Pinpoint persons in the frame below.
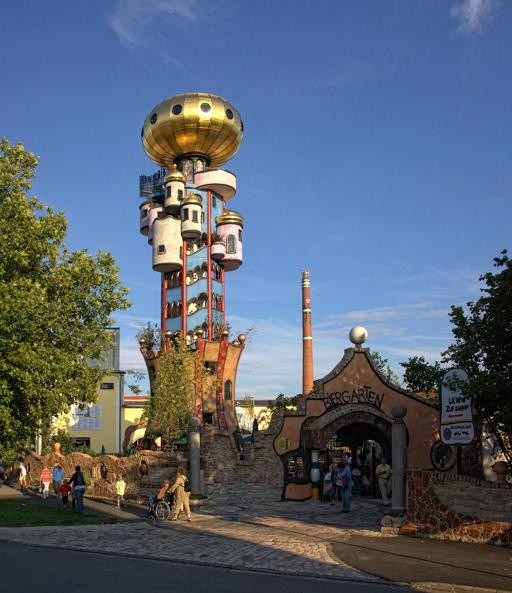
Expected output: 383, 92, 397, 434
126, 440, 144, 455
115, 474, 128, 509
141, 479, 170, 520
0, 462, 27, 491
166, 465, 192, 523
39, 464, 86, 513
331, 446, 392, 512
101, 443, 107, 456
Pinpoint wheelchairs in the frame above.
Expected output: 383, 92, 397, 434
148, 491, 173, 521
